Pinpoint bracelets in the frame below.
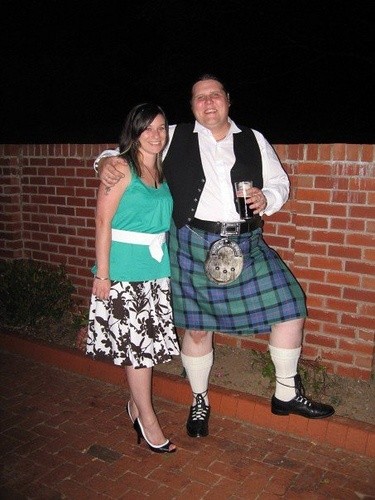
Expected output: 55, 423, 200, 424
95, 275, 110, 280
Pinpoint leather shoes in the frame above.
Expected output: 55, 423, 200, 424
186, 388, 212, 439
270, 395, 336, 419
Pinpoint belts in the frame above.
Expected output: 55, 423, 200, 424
188, 214, 267, 235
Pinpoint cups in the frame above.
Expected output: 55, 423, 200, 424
235, 181, 254, 220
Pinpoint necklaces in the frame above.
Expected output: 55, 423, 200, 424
144, 164, 157, 188
216, 135, 226, 142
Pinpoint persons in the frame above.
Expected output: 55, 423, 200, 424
86, 103, 181, 454
91, 69, 336, 440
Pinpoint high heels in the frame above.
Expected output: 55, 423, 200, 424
126, 400, 134, 424
132, 416, 178, 455
